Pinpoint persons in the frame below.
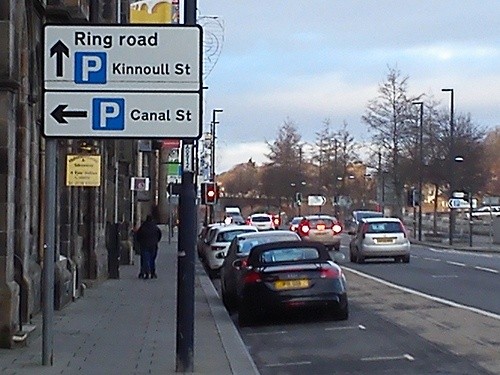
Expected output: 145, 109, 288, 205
133, 216, 162, 280
107, 220, 135, 276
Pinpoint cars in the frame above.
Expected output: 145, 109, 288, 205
297, 215, 342, 250
197, 207, 302, 279
232, 240, 349, 321
465, 206, 500, 218
288, 217, 303, 232
344, 210, 383, 234
215, 230, 305, 313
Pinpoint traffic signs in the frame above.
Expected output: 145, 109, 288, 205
42, 22, 204, 92
42, 90, 203, 141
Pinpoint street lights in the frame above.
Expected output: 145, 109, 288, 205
348, 217, 411, 264
210, 109, 223, 181
412, 101, 424, 242
441, 87, 455, 248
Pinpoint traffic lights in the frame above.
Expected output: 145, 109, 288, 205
200, 182, 219, 206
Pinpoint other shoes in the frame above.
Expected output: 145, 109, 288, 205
151, 273, 155, 279
144, 273, 150, 280
